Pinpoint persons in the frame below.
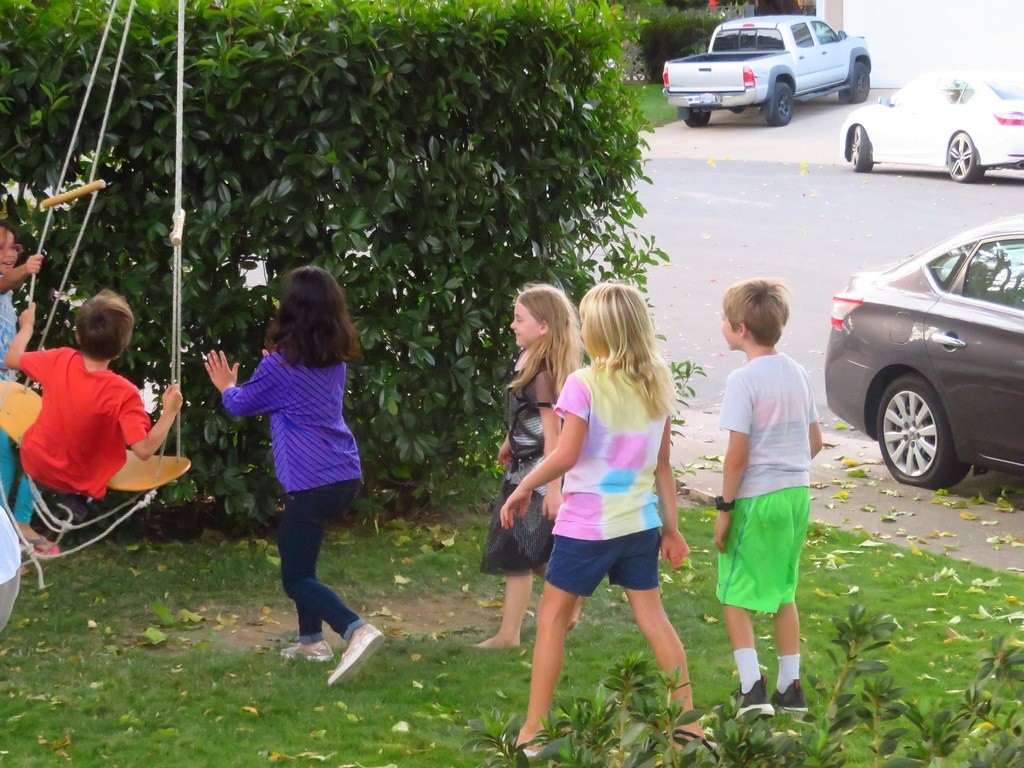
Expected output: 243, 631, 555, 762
205, 267, 385, 685
0, 221, 182, 633
712, 280, 822, 725
472, 283, 716, 768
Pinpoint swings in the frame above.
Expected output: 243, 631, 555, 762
0, 0, 192, 590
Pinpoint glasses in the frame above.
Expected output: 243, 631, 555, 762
0, 244, 24, 255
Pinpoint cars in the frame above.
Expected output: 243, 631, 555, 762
837, 62, 1024, 185
822, 212, 1023, 492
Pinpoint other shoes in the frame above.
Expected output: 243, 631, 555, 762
511, 737, 539, 758
697, 741, 717, 749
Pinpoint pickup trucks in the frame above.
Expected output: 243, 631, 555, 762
660, 13, 873, 128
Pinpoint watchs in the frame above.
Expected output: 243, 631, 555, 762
715, 495, 737, 512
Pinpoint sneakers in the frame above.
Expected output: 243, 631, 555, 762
730, 675, 775, 722
327, 624, 385, 686
769, 679, 808, 712
281, 640, 334, 662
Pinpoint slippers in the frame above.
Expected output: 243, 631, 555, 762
20, 533, 59, 556
